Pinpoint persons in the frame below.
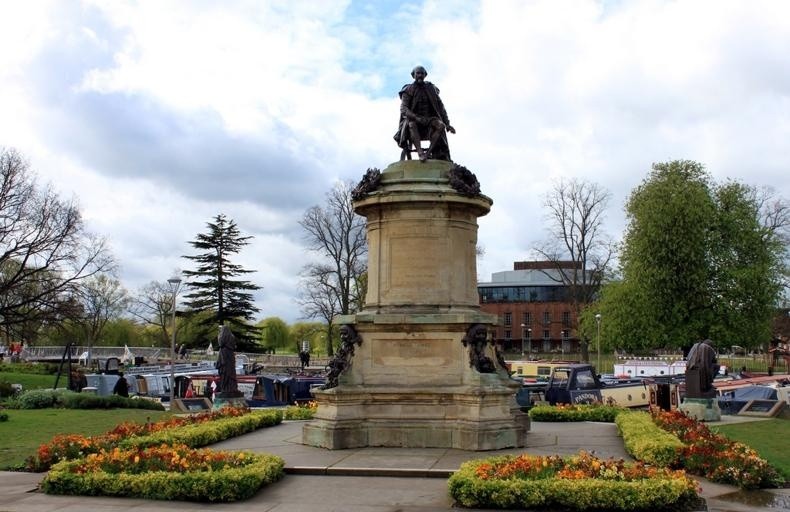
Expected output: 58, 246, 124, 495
175, 343, 179, 359
179, 344, 187, 360
215, 325, 239, 394
69, 367, 87, 393
686, 339, 720, 393
112, 372, 129, 397
299, 350, 311, 372
392, 66, 456, 163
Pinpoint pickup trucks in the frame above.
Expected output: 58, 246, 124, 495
545, 365, 658, 408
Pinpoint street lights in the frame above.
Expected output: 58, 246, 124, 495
167, 276, 182, 413
595, 314, 602, 377
520, 324, 532, 357
560, 332, 565, 360
